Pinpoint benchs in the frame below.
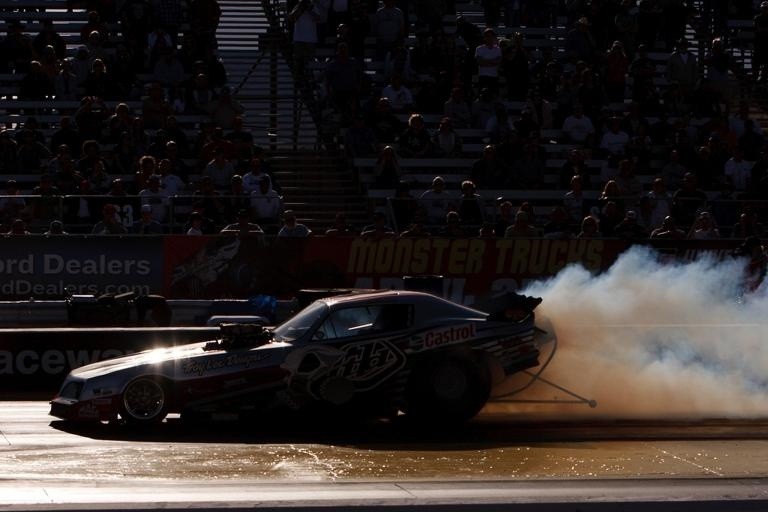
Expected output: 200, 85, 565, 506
1, 0, 288, 232
293, 0, 767, 241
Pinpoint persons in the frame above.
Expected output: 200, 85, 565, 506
1, 0, 765, 242
727, 235, 767, 292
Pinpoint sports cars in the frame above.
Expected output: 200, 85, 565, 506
48, 289, 547, 444
